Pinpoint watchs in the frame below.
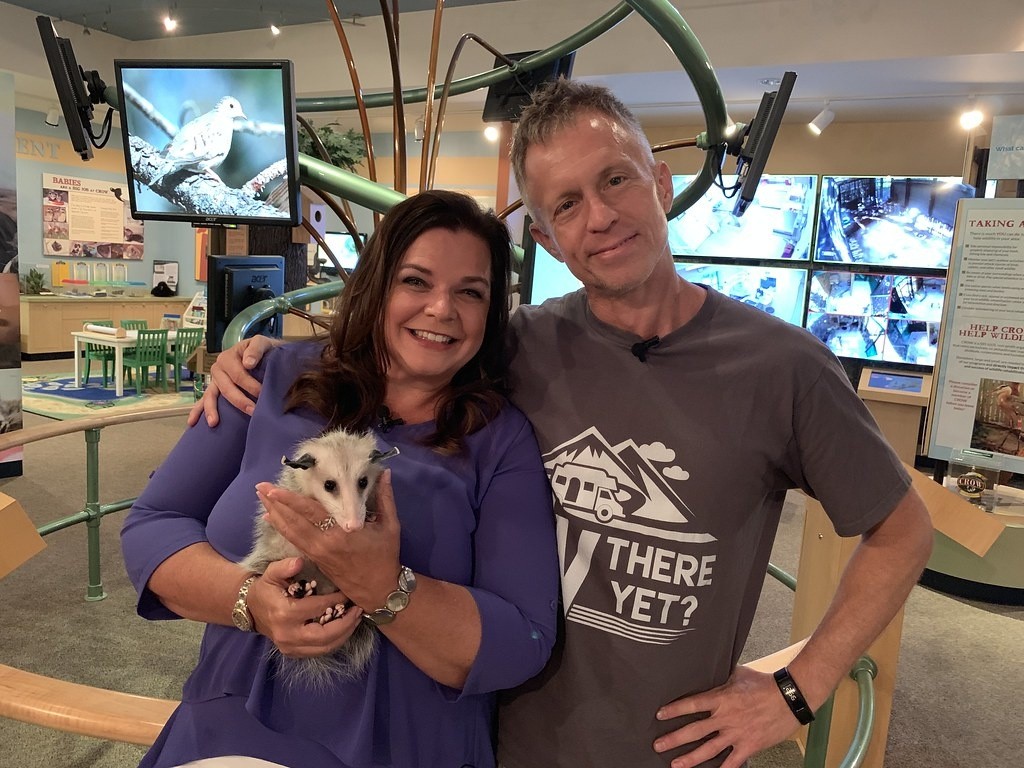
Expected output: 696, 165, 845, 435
229, 574, 259, 635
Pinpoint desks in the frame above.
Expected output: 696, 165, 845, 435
71, 329, 205, 396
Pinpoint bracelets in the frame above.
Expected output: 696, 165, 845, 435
358, 563, 417, 626
773, 667, 816, 727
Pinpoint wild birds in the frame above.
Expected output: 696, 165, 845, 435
148, 96, 247, 189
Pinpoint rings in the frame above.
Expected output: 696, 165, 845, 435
311, 513, 336, 532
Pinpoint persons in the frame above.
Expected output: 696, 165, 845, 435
117, 189, 560, 768
43, 189, 67, 206
185, 82, 934, 768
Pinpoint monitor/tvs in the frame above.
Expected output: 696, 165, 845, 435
318, 232, 366, 272
116, 59, 300, 221
663, 175, 966, 371
36, 15, 90, 161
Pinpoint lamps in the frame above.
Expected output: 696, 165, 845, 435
414, 115, 424, 140
102, 22, 108, 32
726, 114, 736, 135
270, 12, 287, 34
808, 100, 834, 134
82, 15, 90, 35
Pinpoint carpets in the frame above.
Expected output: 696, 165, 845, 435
22, 364, 195, 421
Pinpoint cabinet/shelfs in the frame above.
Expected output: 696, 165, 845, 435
20, 299, 192, 353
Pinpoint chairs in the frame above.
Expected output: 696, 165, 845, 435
84, 320, 204, 396
